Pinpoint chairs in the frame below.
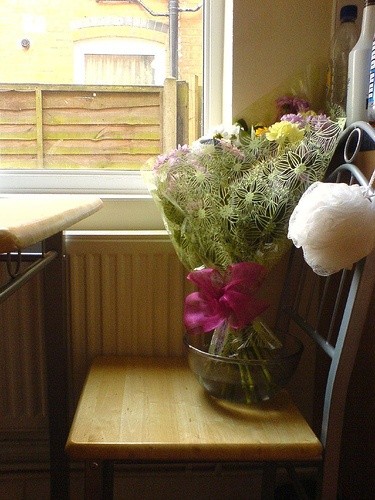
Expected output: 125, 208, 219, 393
64, 118, 375, 500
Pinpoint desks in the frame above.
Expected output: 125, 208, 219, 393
0, 192, 103, 500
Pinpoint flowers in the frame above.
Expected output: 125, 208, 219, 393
140, 56, 347, 411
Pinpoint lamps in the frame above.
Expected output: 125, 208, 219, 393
331, 8, 360, 68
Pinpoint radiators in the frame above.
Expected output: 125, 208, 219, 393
0, 229, 217, 431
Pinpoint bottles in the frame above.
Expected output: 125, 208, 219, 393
348, 3, 374, 127
327, 6, 358, 125
368, 41, 375, 123
